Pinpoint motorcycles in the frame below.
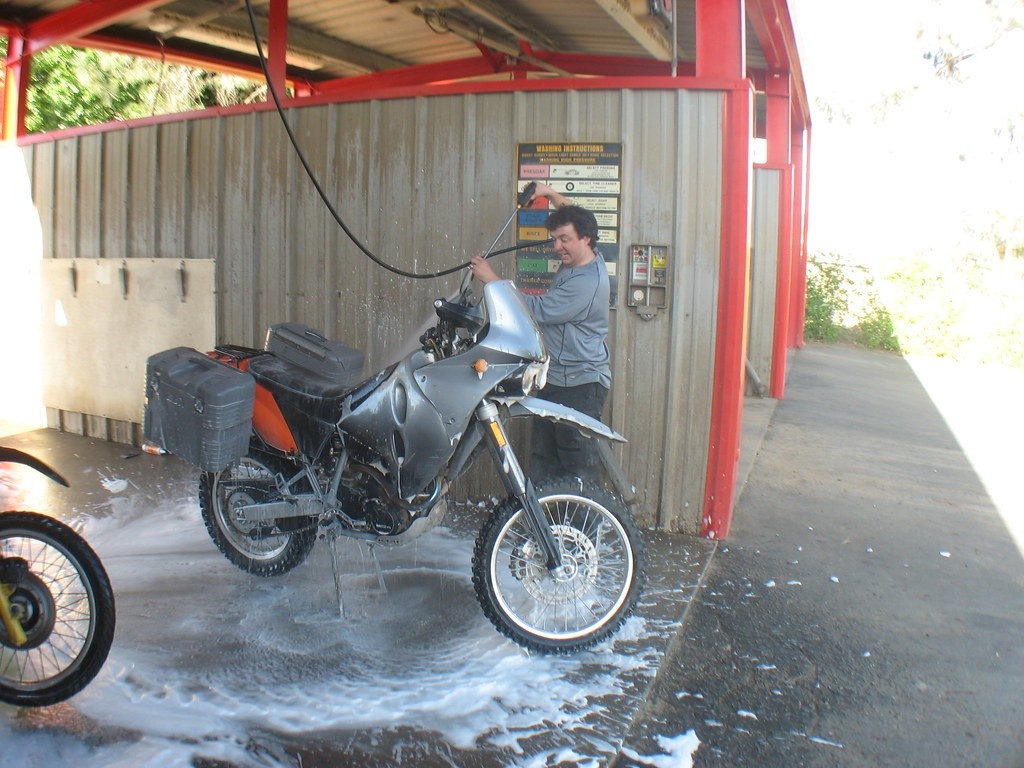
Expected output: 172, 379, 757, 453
143, 281, 647, 658
0, 447, 116, 706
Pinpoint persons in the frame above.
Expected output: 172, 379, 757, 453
470, 179, 612, 565
